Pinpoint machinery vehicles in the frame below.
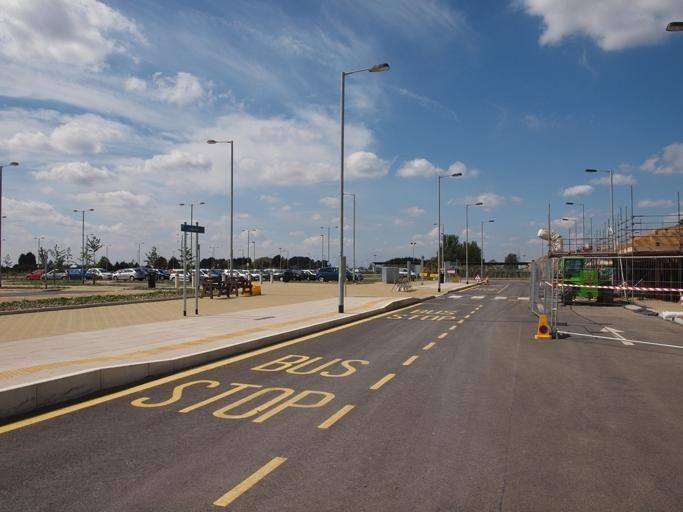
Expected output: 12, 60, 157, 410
559, 257, 616, 305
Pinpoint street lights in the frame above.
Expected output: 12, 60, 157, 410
206, 139, 235, 281
561, 168, 616, 254
73, 209, 94, 274
436, 172, 495, 292
179, 202, 205, 275
338, 63, 390, 313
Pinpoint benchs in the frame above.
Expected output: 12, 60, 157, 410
200, 275, 253, 299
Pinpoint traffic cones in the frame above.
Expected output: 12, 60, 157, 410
534, 314, 552, 341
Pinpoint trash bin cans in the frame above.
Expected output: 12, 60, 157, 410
148, 276, 156, 289
440, 274, 444, 283
